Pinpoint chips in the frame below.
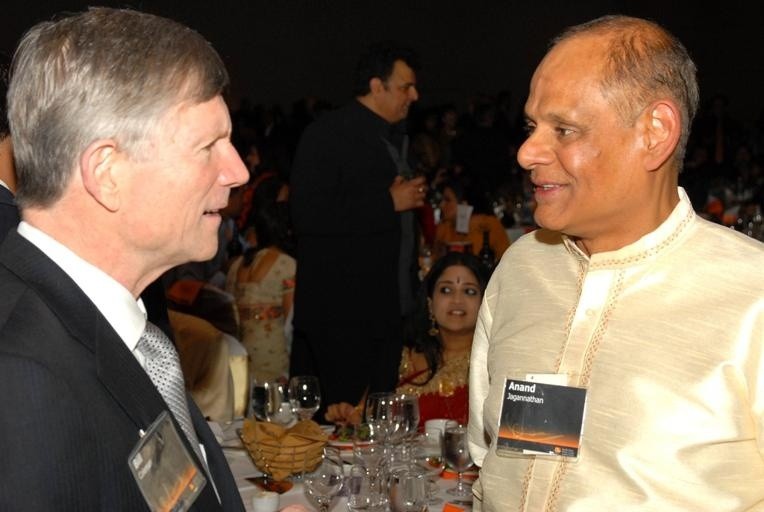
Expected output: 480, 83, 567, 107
242, 418, 329, 482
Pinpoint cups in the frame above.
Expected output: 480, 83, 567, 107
252, 373, 471, 511
397, 165, 422, 184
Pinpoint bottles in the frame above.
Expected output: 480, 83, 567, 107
479, 231, 495, 265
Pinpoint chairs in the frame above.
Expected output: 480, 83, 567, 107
192, 285, 240, 339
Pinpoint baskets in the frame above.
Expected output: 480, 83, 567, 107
236, 428, 326, 480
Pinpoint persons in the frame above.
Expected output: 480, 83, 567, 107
1, 88, 349, 389
391, 90, 763, 441
469, 15, 763, 511
288, 42, 430, 435
0, 5, 252, 511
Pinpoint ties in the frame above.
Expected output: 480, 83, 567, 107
137, 320, 221, 505
